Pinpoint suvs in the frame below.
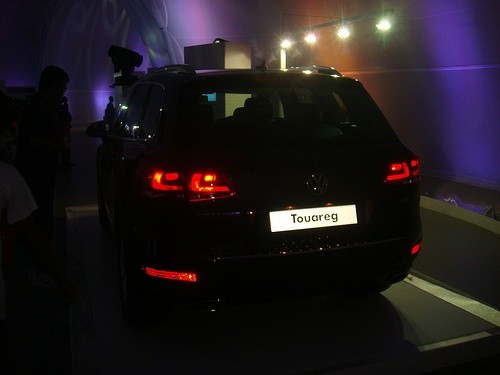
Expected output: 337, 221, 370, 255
84, 64, 423, 325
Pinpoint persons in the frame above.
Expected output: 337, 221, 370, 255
104, 96, 115, 131
0, 162, 42, 319
0, 65, 72, 242
